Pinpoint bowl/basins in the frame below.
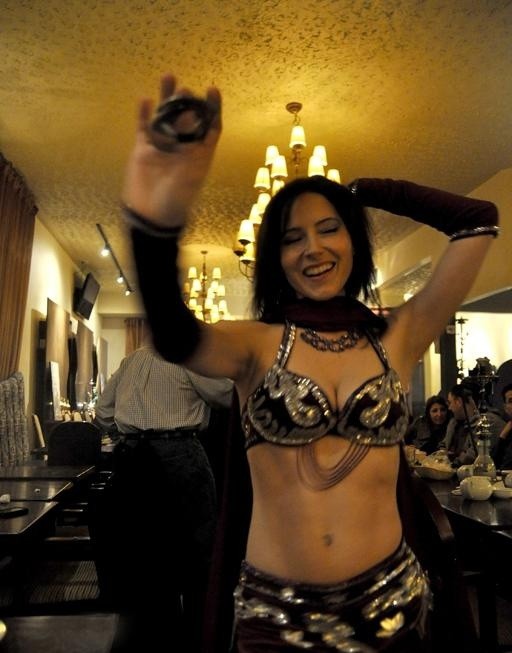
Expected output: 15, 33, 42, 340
490, 486, 511, 498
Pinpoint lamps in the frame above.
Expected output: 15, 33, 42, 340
234, 102, 341, 287
95, 223, 132, 296
182, 250, 233, 326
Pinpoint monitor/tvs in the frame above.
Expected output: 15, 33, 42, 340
74, 272, 101, 320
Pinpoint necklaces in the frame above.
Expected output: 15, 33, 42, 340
301, 326, 365, 354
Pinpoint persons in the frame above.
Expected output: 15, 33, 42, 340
401, 372, 512, 474
92, 344, 235, 652
122, 73, 496, 651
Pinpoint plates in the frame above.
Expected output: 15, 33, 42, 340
412, 465, 455, 479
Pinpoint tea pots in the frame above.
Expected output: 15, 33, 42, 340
459, 476, 493, 502
